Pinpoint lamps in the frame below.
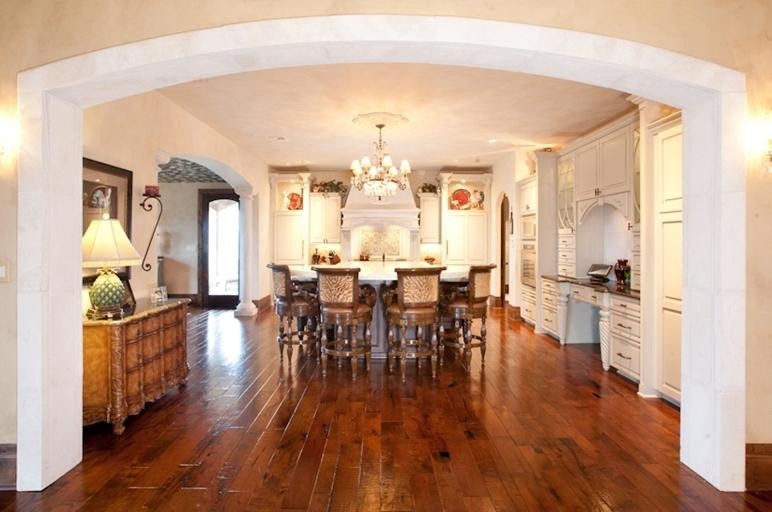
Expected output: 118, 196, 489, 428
83, 217, 144, 321
348, 121, 412, 201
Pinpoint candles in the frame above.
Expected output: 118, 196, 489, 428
144, 185, 161, 195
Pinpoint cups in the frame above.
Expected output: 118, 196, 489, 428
152, 286, 167, 304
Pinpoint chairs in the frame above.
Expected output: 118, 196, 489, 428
266, 263, 497, 383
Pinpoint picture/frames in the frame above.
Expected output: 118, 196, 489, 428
83, 157, 132, 282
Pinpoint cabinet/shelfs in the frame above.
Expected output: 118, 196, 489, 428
310, 192, 341, 245
269, 171, 309, 264
440, 170, 489, 261
416, 193, 440, 245
83, 297, 194, 435
517, 115, 641, 384
